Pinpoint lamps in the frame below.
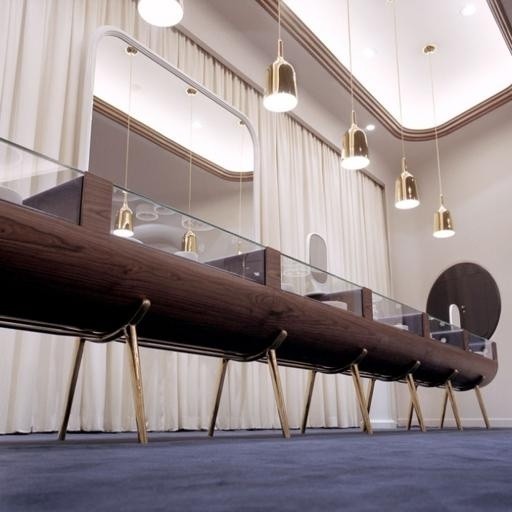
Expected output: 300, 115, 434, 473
235, 121, 248, 259
264, 0, 298, 113
393, 1, 420, 209
136, 0, 184, 28
426, 44, 458, 239
341, 1, 369, 172
114, 47, 139, 238
181, 89, 199, 259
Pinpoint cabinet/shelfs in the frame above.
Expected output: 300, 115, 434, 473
0, 139, 498, 443
